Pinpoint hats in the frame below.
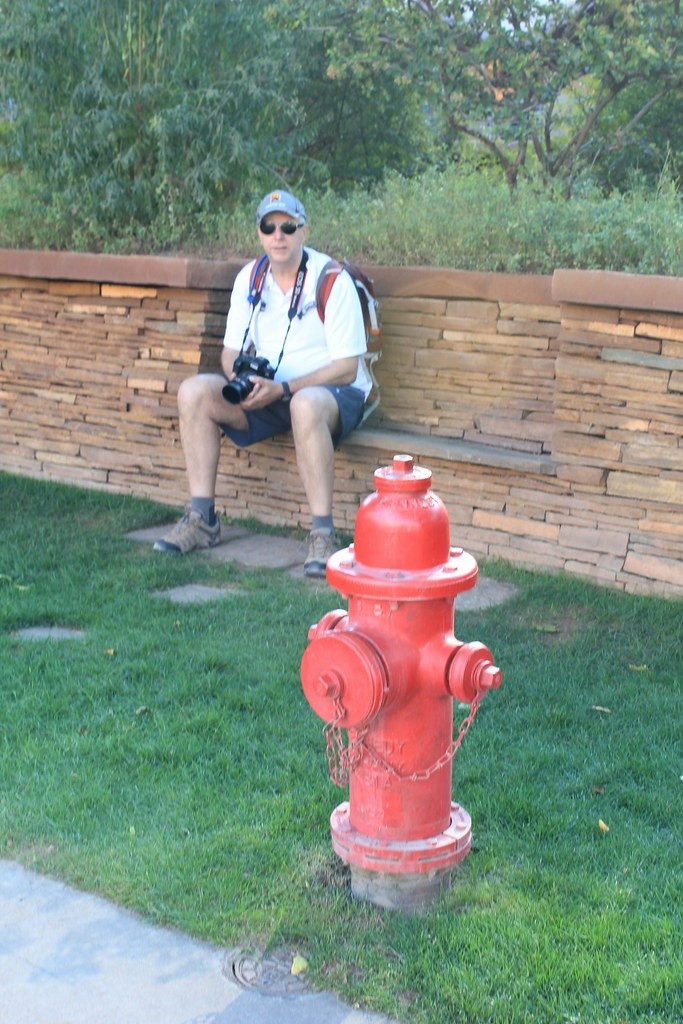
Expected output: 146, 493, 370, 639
255, 189, 306, 225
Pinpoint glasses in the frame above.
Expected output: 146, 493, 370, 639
260, 221, 305, 235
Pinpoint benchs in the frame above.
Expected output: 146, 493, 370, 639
290, 423, 558, 575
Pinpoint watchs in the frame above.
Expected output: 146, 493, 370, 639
281, 381, 293, 402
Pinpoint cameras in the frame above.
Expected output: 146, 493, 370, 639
221, 354, 274, 405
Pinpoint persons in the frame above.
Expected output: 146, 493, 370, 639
152, 188, 374, 578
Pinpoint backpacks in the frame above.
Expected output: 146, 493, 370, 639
248, 254, 382, 364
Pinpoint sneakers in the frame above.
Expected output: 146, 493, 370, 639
296, 527, 343, 578
152, 506, 222, 553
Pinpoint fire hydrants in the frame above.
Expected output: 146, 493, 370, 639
297, 452, 503, 921
